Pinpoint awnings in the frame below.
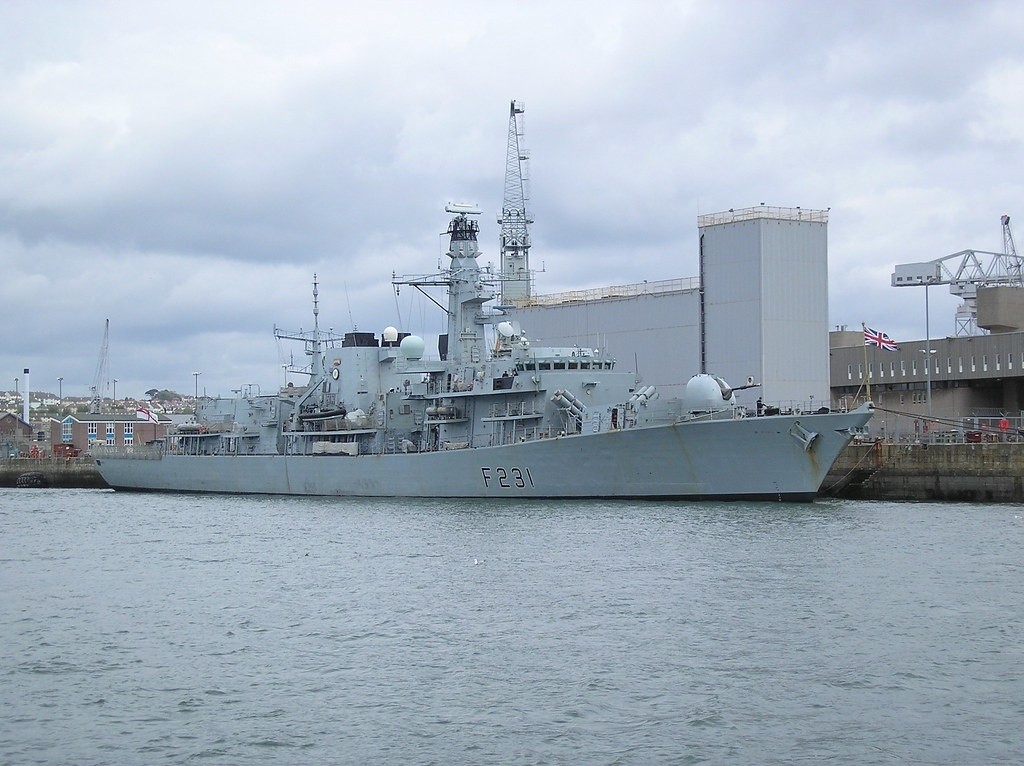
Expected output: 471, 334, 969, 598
863, 327, 897, 352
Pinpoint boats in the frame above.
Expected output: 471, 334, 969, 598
93, 205, 876, 507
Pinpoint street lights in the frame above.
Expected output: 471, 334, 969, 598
112, 378, 118, 403
14, 377, 19, 430
192, 371, 202, 399
57, 377, 64, 422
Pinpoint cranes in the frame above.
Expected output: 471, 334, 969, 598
890, 215, 1024, 339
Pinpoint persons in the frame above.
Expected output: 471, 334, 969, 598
502, 371, 508, 377
757, 397, 763, 417
